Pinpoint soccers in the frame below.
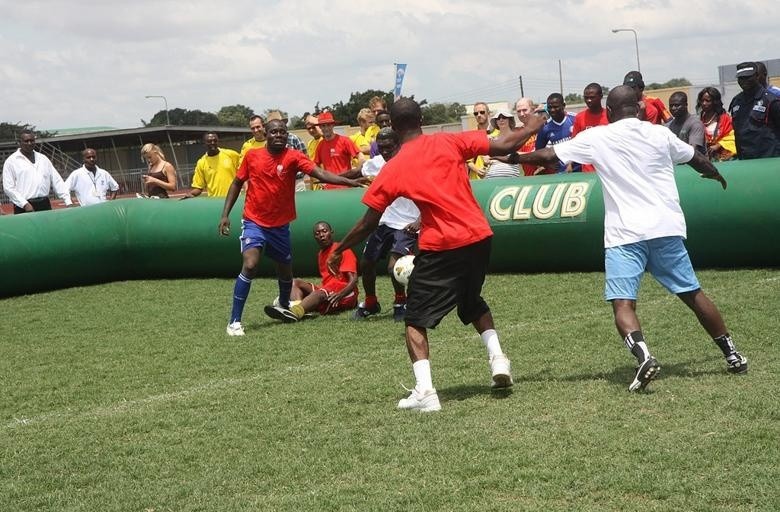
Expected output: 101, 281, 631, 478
393, 253, 415, 284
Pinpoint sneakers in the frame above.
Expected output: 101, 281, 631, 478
726, 355, 748, 375
626, 355, 661, 393
263, 305, 298, 324
392, 300, 407, 323
226, 320, 246, 337
351, 302, 381, 322
397, 386, 441, 413
488, 357, 514, 390
273, 296, 292, 310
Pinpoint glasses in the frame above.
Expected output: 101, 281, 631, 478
473, 111, 487, 115
495, 114, 509, 120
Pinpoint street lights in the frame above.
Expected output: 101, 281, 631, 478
145, 95, 170, 126
611, 28, 641, 72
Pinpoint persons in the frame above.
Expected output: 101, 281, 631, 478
623, 62, 780, 165
181, 95, 392, 200
2, 130, 64, 214
326, 97, 549, 412
65, 147, 120, 208
264, 220, 360, 322
140, 143, 178, 199
218, 118, 371, 336
488, 84, 748, 391
310, 125, 420, 322
465, 83, 610, 179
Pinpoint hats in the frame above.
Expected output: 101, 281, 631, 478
734, 61, 758, 78
489, 108, 517, 130
312, 112, 338, 127
263, 109, 289, 126
622, 71, 642, 89
